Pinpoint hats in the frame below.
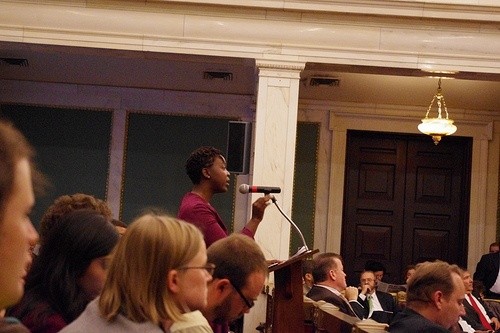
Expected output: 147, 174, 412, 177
467, 293, 495, 333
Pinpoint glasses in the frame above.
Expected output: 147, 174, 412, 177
176, 263, 216, 276
229, 275, 255, 309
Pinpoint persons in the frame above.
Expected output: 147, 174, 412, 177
0, 121, 500, 333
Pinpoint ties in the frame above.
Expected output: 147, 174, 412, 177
339, 294, 359, 318
368, 296, 373, 313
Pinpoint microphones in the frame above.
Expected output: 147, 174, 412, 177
238, 184, 282, 197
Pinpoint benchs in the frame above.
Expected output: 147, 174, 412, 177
261, 283, 317, 333
315, 302, 389, 333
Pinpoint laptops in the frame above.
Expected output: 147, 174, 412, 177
369, 310, 394, 325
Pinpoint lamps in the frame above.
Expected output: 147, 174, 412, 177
417, 79, 457, 145
